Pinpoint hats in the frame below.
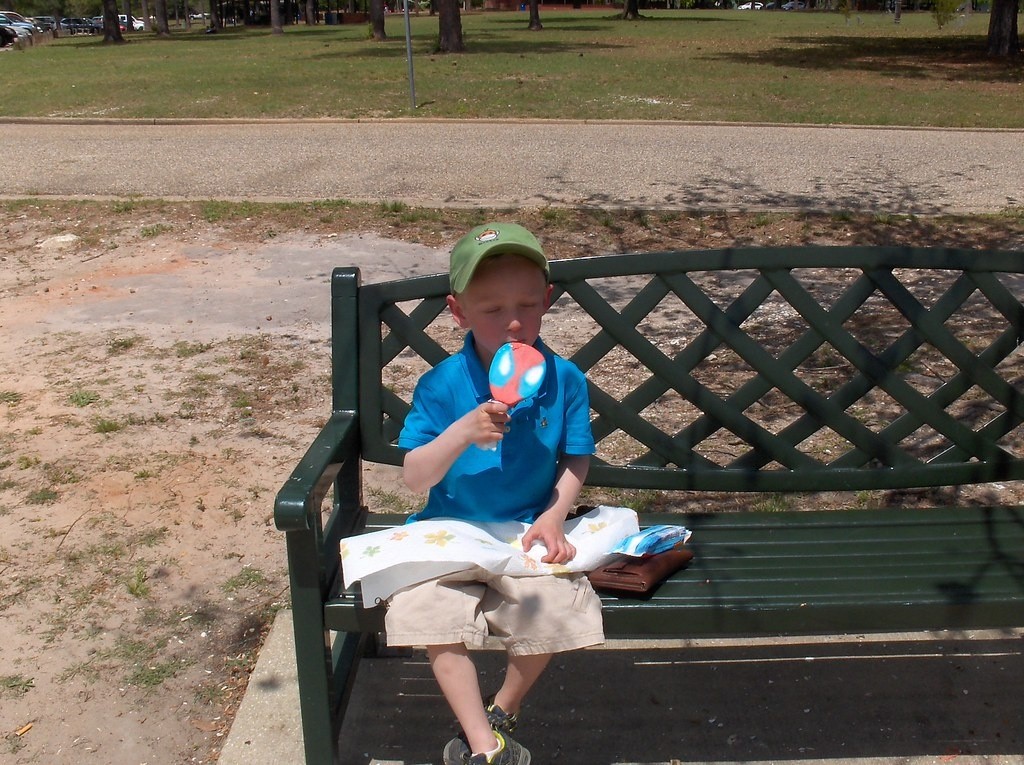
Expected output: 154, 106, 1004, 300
449, 222, 550, 296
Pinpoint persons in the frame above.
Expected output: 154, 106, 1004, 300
520, 3, 525, 11
384, 6, 388, 13
386, 222, 604, 765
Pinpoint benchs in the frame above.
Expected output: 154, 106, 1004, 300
272, 243, 1023, 765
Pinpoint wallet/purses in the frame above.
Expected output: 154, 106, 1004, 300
588, 548, 693, 592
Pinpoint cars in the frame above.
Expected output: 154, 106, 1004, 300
188, 12, 210, 20
738, 1, 764, 10
760, 2, 782, 10
0, 10, 157, 48
780, 2, 805, 12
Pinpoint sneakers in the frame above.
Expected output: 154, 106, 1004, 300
443, 704, 519, 765
458, 728, 532, 765
